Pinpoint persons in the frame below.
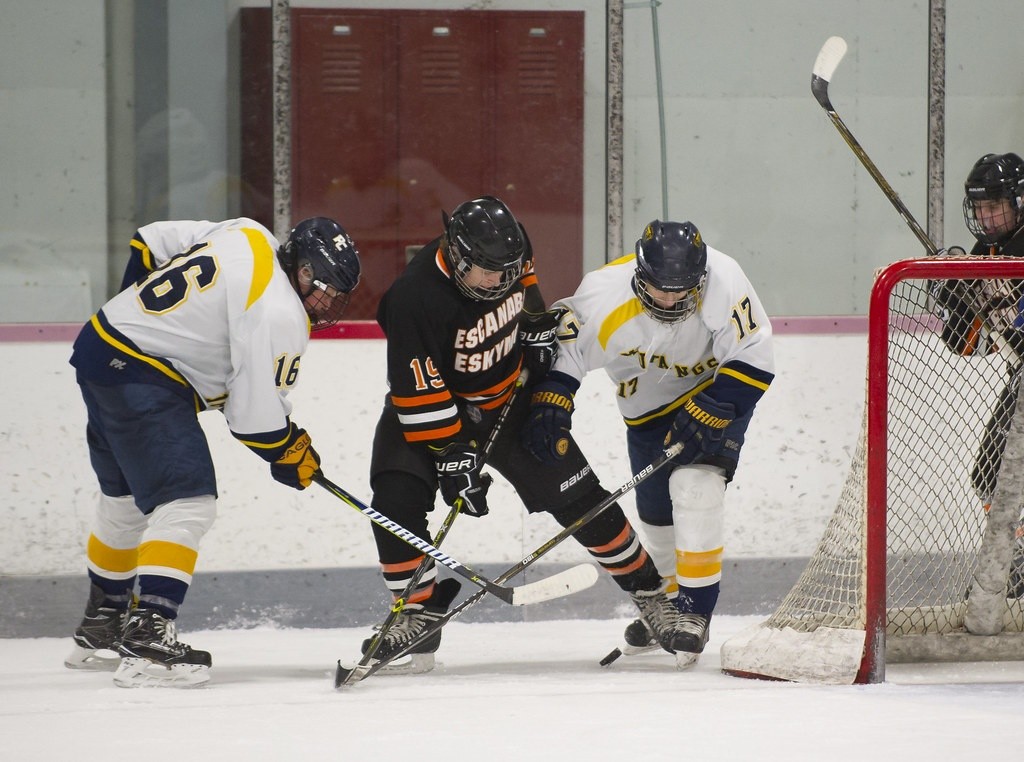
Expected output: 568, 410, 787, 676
67, 215, 360, 691
522, 219, 775, 670
928, 153, 1024, 634
362, 195, 679, 676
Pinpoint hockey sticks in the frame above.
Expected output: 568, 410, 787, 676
810, 34, 1022, 371
334, 432, 683, 685
310, 475, 600, 608
334, 364, 535, 692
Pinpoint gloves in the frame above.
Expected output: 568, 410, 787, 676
429, 446, 492, 516
941, 310, 995, 356
924, 246, 985, 305
526, 408, 572, 462
664, 396, 735, 465
272, 429, 320, 492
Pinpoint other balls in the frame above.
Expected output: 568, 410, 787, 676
599, 646, 622, 667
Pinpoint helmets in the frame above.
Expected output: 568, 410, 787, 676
288, 216, 361, 289
966, 152, 1023, 198
450, 195, 527, 270
634, 221, 706, 290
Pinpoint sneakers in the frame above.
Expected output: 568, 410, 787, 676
63, 579, 212, 685
675, 588, 711, 666
627, 582, 682, 656
623, 610, 656, 656
361, 579, 461, 676
